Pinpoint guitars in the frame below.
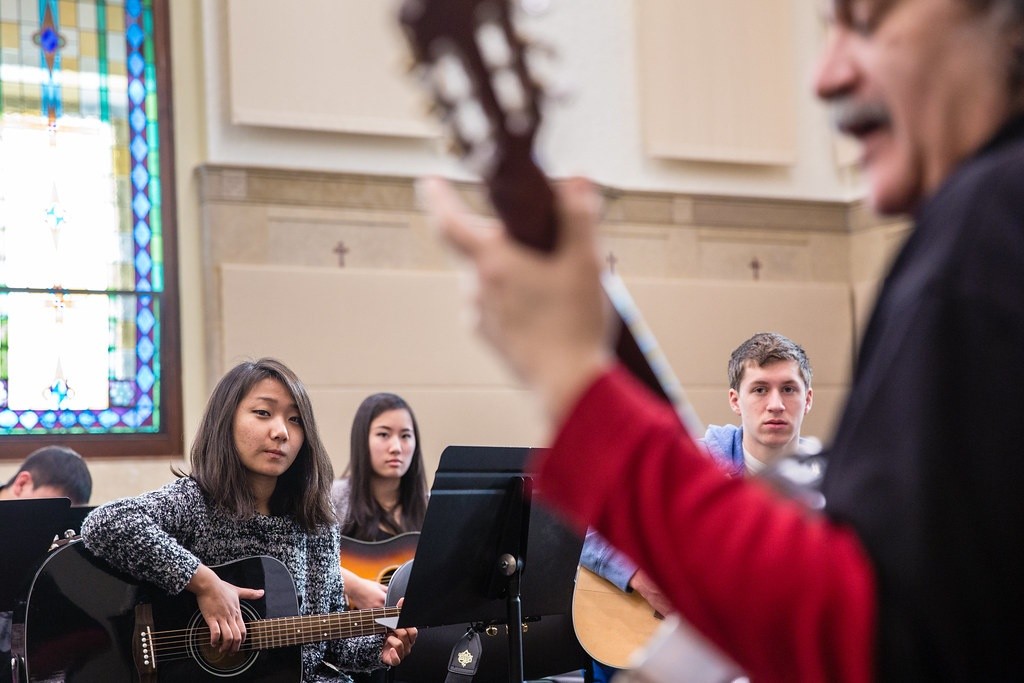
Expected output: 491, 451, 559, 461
340, 531, 423, 611
9, 528, 404, 683
396, 0, 688, 427
570, 560, 669, 670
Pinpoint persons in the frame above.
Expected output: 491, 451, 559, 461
0, 445, 92, 683
80, 357, 418, 683
332, 392, 431, 610
418, 1, 1023, 681
580, 334, 830, 622
